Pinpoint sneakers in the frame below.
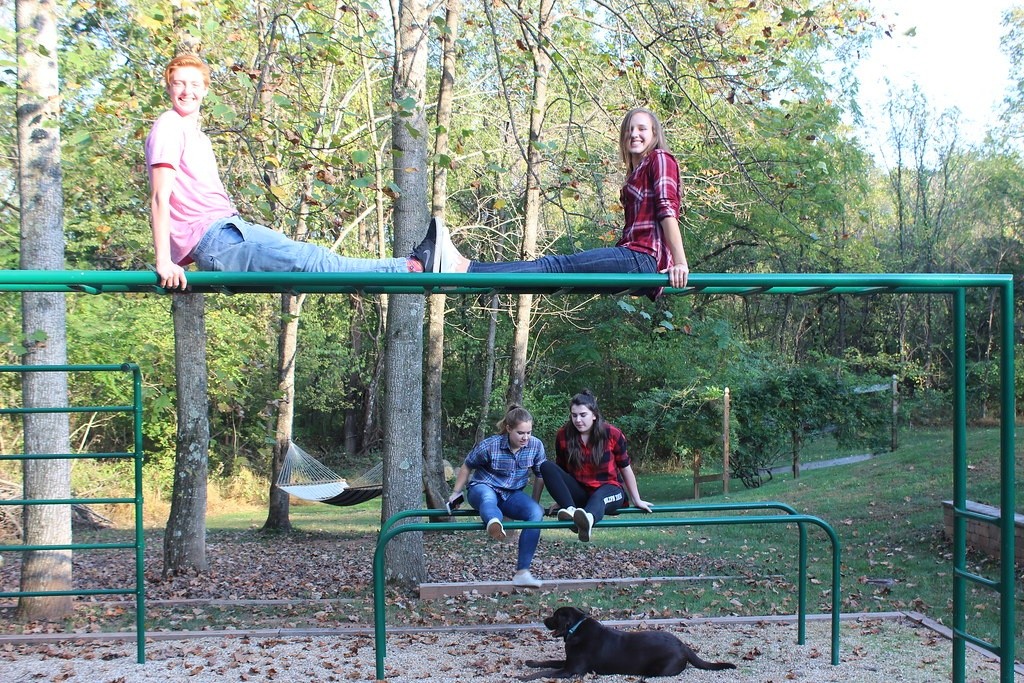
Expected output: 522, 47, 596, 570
410, 217, 443, 274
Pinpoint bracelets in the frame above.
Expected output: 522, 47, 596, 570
453, 490, 461, 494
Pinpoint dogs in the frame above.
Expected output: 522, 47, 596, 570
518, 607, 738, 683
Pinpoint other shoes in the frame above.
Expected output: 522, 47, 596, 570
558, 507, 578, 534
486, 518, 507, 542
439, 226, 461, 273
512, 571, 542, 588
575, 508, 593, 542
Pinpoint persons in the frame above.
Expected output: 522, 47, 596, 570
440, 108, 691, 290
145, 55, 442, 291
540, 388, 655, 542
449, 402, 547, 588
